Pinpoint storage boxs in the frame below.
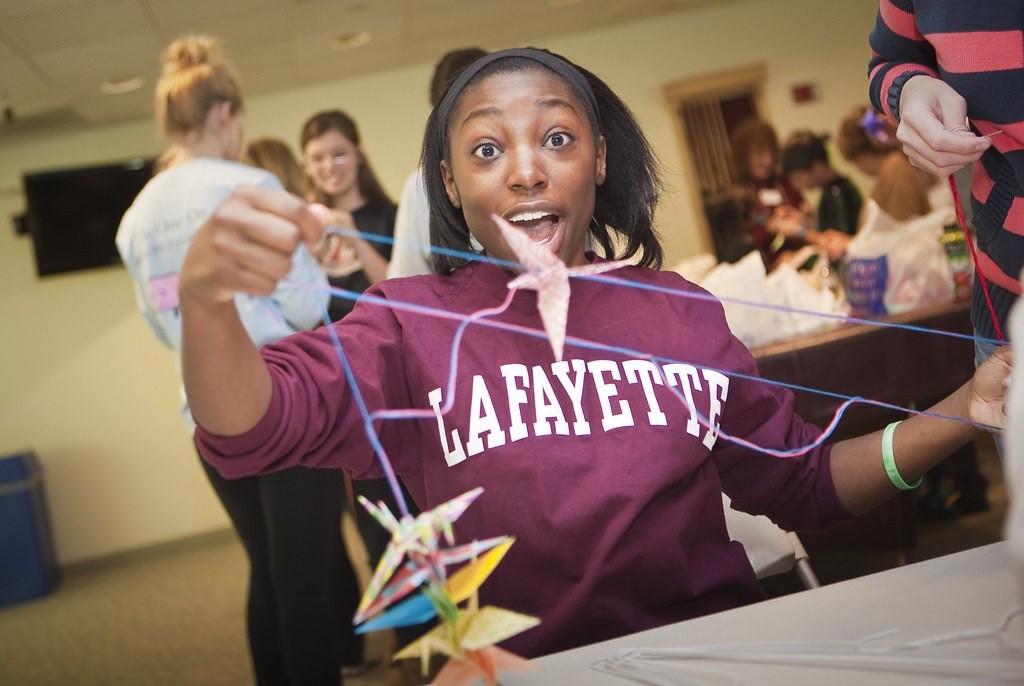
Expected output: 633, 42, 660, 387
0, 449, 66, 610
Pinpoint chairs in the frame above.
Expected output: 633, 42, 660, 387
720, 490, 822, 591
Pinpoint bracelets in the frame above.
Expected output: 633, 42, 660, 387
881, 420, 923, 491
887, 70, 928, 121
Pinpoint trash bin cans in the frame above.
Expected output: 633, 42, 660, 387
0, 447, 59, 609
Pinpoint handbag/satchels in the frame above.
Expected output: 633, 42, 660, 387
709, 250, 851, 350
842, 197, 957, 315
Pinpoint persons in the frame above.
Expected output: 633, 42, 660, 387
868, 0, 1024, 455
116, 37, 345, 686
239, 136, 317, 204
389, 47, 491, 281
722, 103, 990, 523
300, 108, 399, 328
178, 46, 1016, 662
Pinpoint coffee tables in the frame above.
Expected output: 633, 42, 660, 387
748, 293, 972, 361
468, 537, 1024, 686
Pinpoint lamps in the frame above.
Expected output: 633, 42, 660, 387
100, 74, 147, 94
331, 30, 376, 50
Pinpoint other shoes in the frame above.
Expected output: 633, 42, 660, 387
341, 661, 373, 676
915, 474, 989, 520
